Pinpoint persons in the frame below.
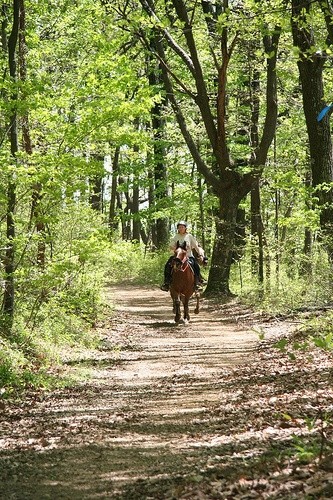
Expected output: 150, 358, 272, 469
160, 221, 204, 292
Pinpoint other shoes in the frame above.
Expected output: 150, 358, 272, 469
193, 285, 204, 291
161, 284, 169, 292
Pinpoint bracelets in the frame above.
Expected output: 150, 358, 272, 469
198, 255, 202, 257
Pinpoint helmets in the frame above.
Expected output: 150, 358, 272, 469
177, 220, 187, 227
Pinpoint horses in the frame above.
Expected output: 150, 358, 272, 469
168, 240, 203, 324
